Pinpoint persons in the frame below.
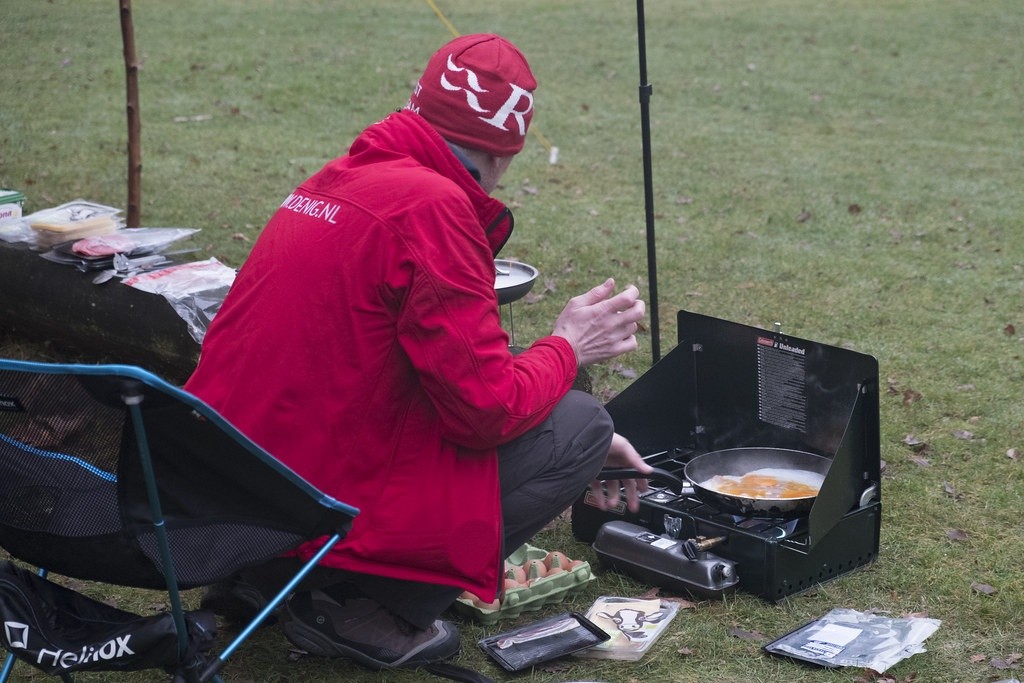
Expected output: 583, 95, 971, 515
178, 32, 653, 669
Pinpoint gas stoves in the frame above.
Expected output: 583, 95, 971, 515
565, 444, 882, 605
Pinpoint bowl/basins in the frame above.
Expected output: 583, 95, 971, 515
0, 186, 184, 327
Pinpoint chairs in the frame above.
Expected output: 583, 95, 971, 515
2, 353, 361, 683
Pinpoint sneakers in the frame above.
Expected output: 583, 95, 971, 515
195, 572, 284, 624
283, 592, 464, 672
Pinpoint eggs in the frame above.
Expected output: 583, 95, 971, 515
698, 468, 825, 500
462, 552, 583, 610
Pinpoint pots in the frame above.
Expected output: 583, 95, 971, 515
596, 444, 834, 516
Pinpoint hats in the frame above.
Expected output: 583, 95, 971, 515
404, 34, 536, 158
